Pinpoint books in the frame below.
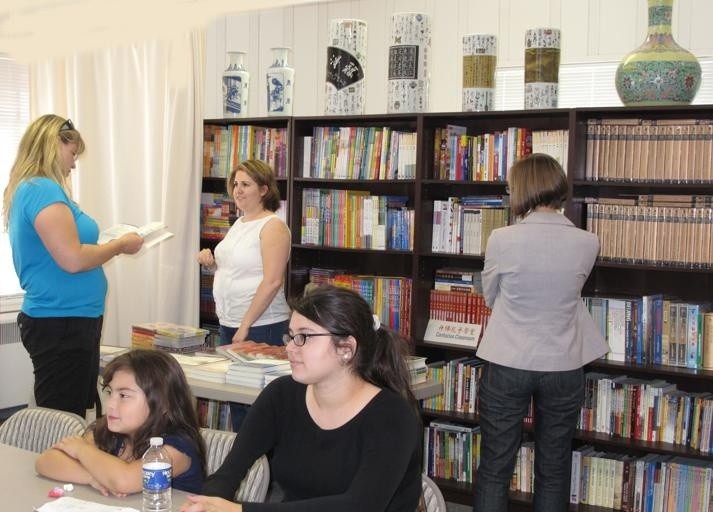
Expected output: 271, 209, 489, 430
126, 318, 200, 400
95, 222, 173, 257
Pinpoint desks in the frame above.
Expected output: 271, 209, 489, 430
87, 340, 443, 411
2, 443, 209, 511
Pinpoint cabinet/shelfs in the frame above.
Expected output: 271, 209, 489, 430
287, 103, 712, 512
199, 116, 291, 341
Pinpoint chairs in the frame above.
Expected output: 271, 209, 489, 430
1, 405, 86, 457
415, 471, 446, 512
199, 428, 269, 503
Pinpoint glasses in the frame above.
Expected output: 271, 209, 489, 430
58, 118, 75, 132
281, 329, 347, 347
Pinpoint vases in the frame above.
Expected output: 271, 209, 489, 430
523, 26, 560, 109
460, 36, 496, 112
384, 10, 429, 114
324, 17, 366, 114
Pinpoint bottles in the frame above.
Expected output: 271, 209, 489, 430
141, 435, 173, 511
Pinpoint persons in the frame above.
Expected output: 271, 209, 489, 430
3, 116, 145, 414
33, 351, 207, 497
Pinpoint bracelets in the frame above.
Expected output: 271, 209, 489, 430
108, 238, 118, 257
